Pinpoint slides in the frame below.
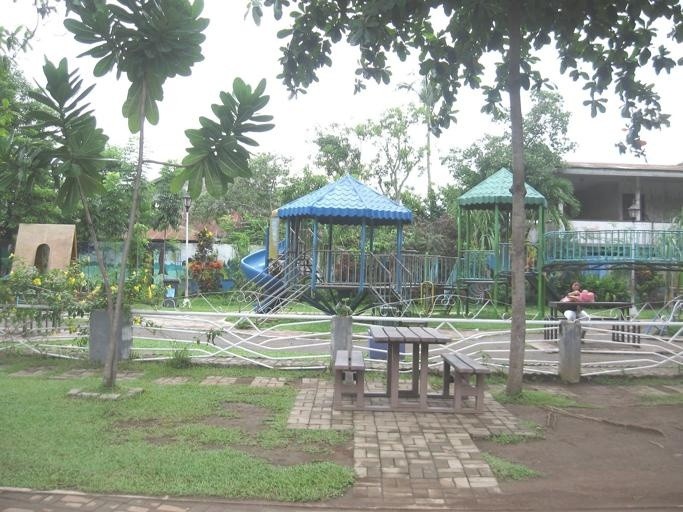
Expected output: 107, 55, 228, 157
444, 256, 463, 314
240, 238, 286, 315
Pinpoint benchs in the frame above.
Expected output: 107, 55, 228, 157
542, 316, 641, 345
440, 353, 490, 414
336, 345, 364, 410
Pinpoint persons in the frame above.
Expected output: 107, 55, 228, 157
558, 281, 590, 344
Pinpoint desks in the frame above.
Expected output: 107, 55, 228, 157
550, 300, 632, 345
368, 324, 451, 414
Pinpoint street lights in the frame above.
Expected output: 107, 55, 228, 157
627, 199, 641, 316
182, 192, 192, 308
